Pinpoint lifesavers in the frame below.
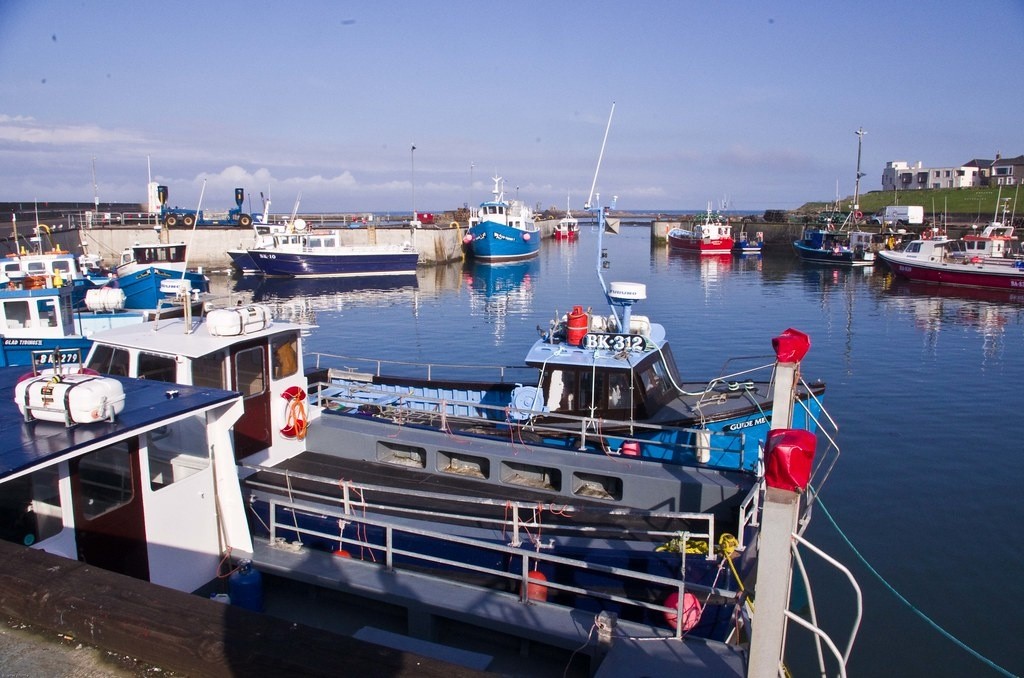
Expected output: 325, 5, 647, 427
165, 213, 179, 227
238, 214, 253, 228
20, 274, 59, 291
44, 250, 70, 255
276, 385, 310, 441
35, 224, 51, 239
4, 252, 35, 258
182, 213, 196, 228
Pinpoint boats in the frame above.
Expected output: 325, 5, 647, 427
226, 184, 339, 273
552, 197, 580, 238
247, 191, 419, 278
947, 184, 1023, 266
731, 232, 764, 251
878, 196, 1024, 291
877, 276, 1024, 375
794, 178, 876, 264
0, 179, 862, 678
463, 174, 541, 261
462, 254, 540, 346
304, 101, 826, 474
668, 200, 733, 252
249, 274, 419, 338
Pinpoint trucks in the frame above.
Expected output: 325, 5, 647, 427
157, 185, 252, 228
871, 206, 924, 227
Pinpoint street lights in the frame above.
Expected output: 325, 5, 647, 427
411, 146, 416, 209
855, 127, 868, 205
